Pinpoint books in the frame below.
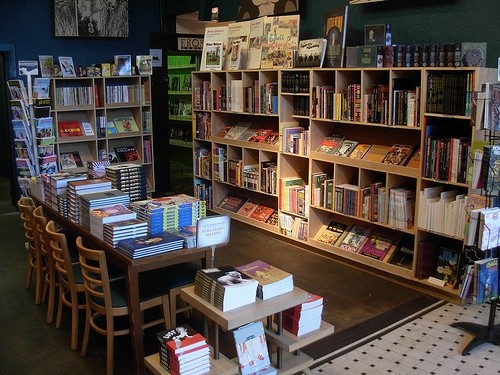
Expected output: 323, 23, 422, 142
56, 87, 93, 106
40, 55, 77, 78
94, 83, 99, 108
14, 139, 87, 213
58, 121, 94, 137
67, 163, 206, 260
278, 70, 500, 303
156, 259, 323, 375
169, 74, 192, 142
200, 15, 300, 70
106, 85, 140, 104
102, 55, 152, 75
295, 6, 486, 68
7, 78, 57, 138
96, 112, 142, 163
193, 80, 278, 227
142, 78, 152, 163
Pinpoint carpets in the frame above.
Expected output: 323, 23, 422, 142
0, 206, 453, 375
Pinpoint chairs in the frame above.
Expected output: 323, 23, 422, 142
17, 194, 207, 375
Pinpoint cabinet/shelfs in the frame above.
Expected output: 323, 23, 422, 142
7, 53, 500, 375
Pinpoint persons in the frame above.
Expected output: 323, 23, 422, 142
13, 87, 56, 174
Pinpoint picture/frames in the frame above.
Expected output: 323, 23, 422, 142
50, 0, 133, 40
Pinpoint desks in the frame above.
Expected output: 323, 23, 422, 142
27, 189, 214, 375
